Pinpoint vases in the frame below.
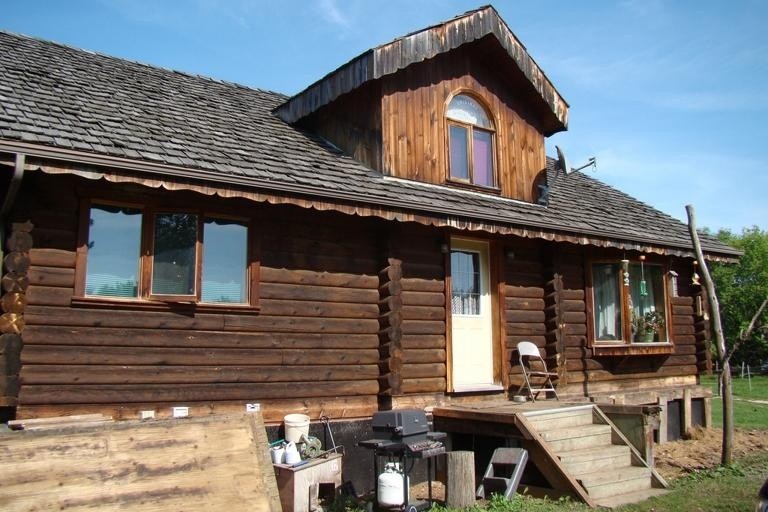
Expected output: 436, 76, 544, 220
634, 329, 655, 342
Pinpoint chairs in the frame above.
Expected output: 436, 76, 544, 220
513, 340, 561, 403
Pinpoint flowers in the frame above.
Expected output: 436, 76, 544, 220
635, 310, 664, 332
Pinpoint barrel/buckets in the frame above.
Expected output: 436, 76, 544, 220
284, 413, 311, 443
